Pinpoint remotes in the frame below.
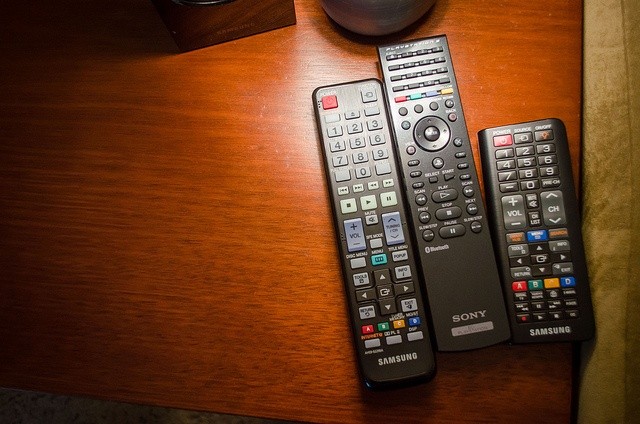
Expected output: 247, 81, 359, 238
377, 34, 511, 353
478, 118, 596, 345
311, 77, 436, 390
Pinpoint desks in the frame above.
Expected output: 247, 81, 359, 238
0, 0, 583, 423
